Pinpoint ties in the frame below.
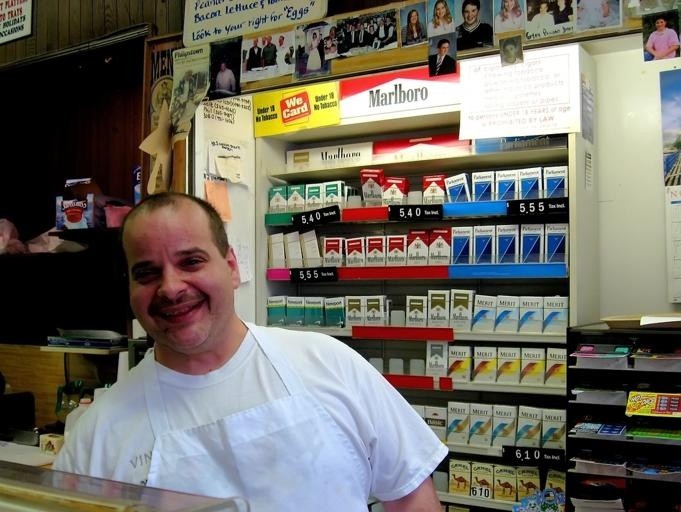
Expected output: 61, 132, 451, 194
434, 58, 443, 74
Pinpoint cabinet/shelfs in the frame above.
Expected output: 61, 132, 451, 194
193, 42, 603, 511
565, 319, 681, 512
0, 459, 240, 511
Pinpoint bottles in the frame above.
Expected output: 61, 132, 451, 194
64, 397, 93, 440
132, 164, 141, 208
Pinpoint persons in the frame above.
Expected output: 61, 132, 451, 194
401, 0, 616, 77
242, 17, 397, 76
216, 64, 235, 92
645, 15, 680, 60
49, 191, 450, 512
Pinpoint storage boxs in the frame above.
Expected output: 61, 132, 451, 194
55, 176, 125, 231
